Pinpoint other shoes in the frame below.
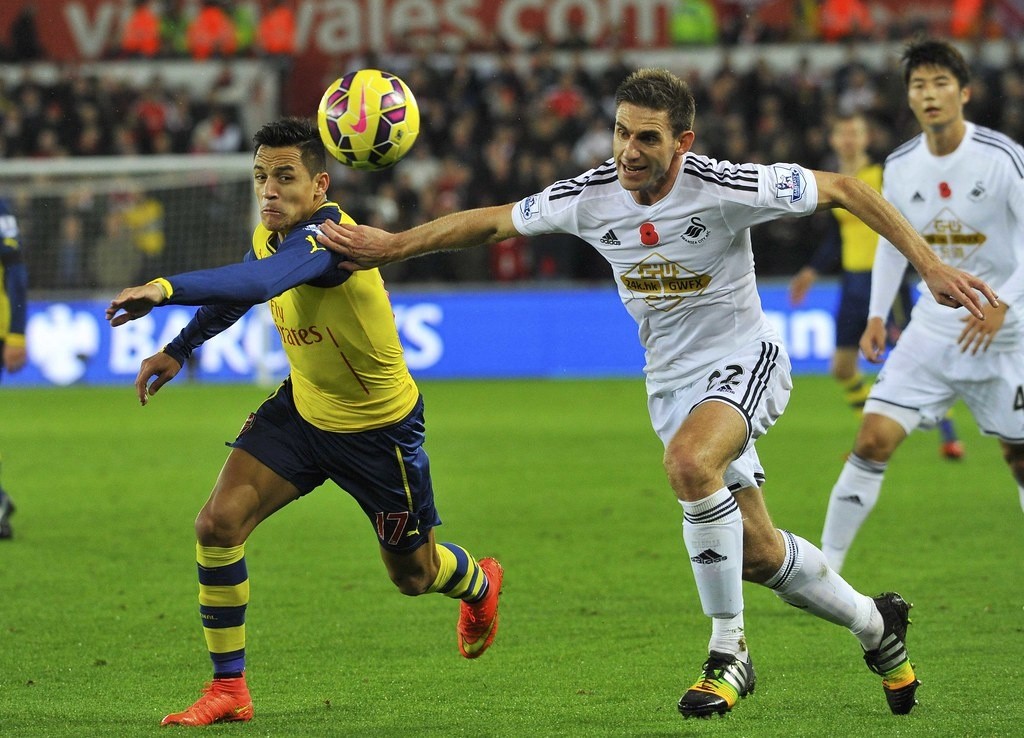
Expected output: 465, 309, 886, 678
943, 440, 962, 461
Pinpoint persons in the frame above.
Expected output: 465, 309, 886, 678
315, 67, 1000, 718
819, 38, 1023, 577
0, 180, 28, 541
0, 0, 1024, 288
789, 108, 963, 461
103, 117, 504, 724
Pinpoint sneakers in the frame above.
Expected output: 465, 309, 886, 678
678, 651, 756, 717
457, 557, 504, 659
160, 672, 253, 726
861, 594, 922, 715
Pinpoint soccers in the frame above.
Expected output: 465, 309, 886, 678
316, 64, 421, 169
